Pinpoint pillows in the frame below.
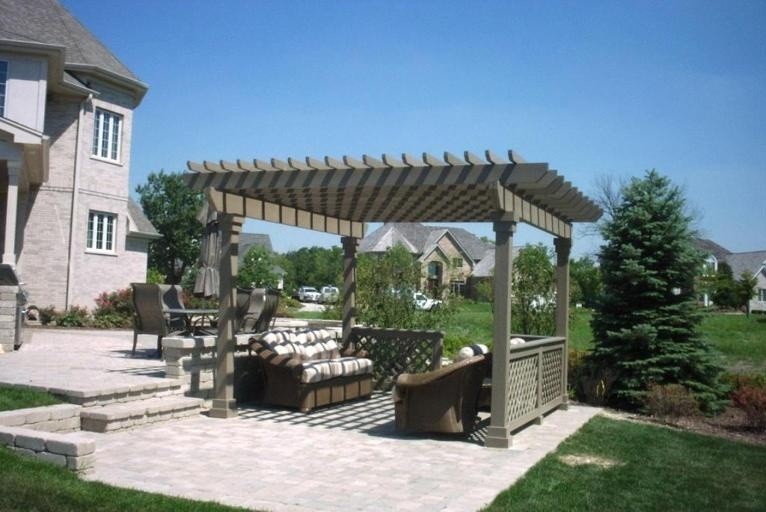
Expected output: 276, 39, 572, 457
455, 343, 488, 362
510, 338, 525, 344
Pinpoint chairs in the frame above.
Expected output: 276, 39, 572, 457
131, 283, 282, 359
478, 378, 492, 409
393, 353, 492, 436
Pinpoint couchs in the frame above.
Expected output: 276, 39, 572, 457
249, 327, 374, 413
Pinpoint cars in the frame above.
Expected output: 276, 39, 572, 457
298, 285, 339, 304
413, 293, 442, 311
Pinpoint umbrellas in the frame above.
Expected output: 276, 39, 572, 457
188, 201, 228, 324
